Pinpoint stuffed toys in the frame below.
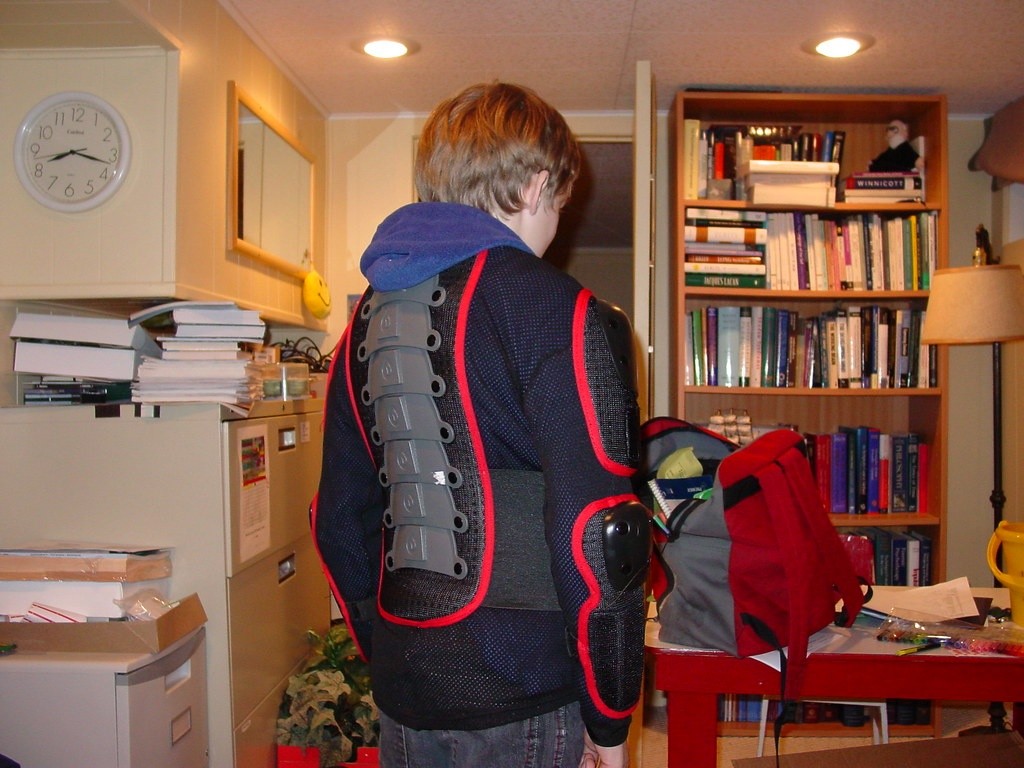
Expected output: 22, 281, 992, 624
866, 120, 924, 173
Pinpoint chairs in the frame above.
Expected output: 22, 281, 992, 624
756, 535, 888, 758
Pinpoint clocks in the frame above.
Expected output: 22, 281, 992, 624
13, 90, 131, 213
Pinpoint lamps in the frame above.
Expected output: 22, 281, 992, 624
922, 247, 1024, 624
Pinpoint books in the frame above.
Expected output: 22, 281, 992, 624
716, 694, 930, 727
647, 476, 713, 534
682, 119, 926, 203
779, 422, 932, 587
130, 301, 266, 417
684, 298, 937, 389
684, 207, 939, 291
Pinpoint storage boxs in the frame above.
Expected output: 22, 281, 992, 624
0, 580, 166, 618
10, 312, 161, 381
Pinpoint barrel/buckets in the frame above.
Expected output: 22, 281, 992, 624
987, 521, 1024, 626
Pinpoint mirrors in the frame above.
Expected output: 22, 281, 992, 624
226, 80, 317, 279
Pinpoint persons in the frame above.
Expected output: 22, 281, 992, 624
310, 83, 652, 768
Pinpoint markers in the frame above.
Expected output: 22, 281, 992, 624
876, 621, 1024, 657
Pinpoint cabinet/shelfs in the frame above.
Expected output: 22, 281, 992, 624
668, 92, 949, 585
0, 408, 332, 768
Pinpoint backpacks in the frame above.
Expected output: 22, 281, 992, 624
635, 416, 874, 705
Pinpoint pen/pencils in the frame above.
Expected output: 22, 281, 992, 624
897, 641, 942, 657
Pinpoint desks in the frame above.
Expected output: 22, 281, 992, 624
644, 584, 1024, 768
